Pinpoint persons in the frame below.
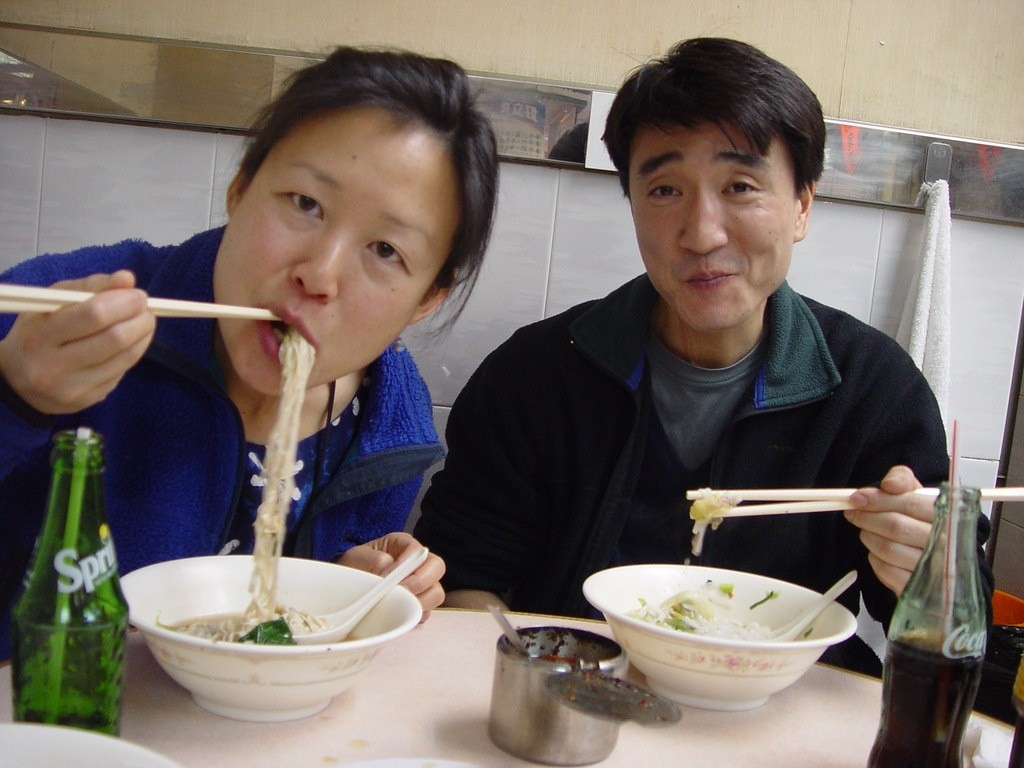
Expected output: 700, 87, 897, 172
1, 48, 501, 667
409, 38, 997, 678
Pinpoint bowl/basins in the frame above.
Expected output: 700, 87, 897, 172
120, 555, 422, 722
583, 564, 857, 709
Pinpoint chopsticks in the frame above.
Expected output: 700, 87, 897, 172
0, 283, 283, 320
687, 487, 1024, 519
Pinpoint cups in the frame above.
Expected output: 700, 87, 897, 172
490, 626, 682, 765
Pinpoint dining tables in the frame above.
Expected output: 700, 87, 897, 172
0, 609, 1016, 768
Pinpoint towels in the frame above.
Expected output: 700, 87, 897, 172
887, 179, 952, 458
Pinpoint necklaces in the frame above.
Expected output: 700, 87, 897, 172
304, 383, 336, 506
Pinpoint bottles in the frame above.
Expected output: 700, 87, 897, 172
11, 431, 129, 735
867, 480, 987, 768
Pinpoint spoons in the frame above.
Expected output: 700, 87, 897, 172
770, 570, 857, 642
286, 548, 428, 645
